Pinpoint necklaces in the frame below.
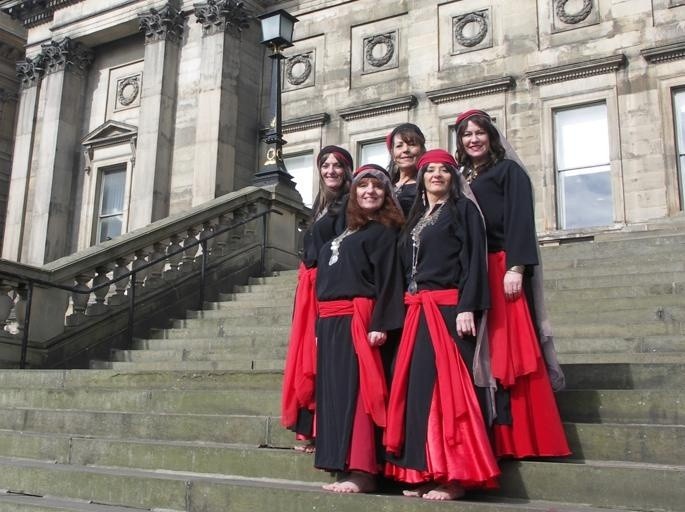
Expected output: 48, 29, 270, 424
393, 176, 411, 197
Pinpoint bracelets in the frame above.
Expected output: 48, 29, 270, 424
506, 264, 528, 273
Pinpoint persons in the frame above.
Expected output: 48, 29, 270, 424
280, 146, 355, 453
311, 165, 405, 494
446, 108, 575, 460
380, 119, 433, 214
379, 149, 506, 501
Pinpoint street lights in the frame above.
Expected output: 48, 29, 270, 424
251, 7, 300, 192
